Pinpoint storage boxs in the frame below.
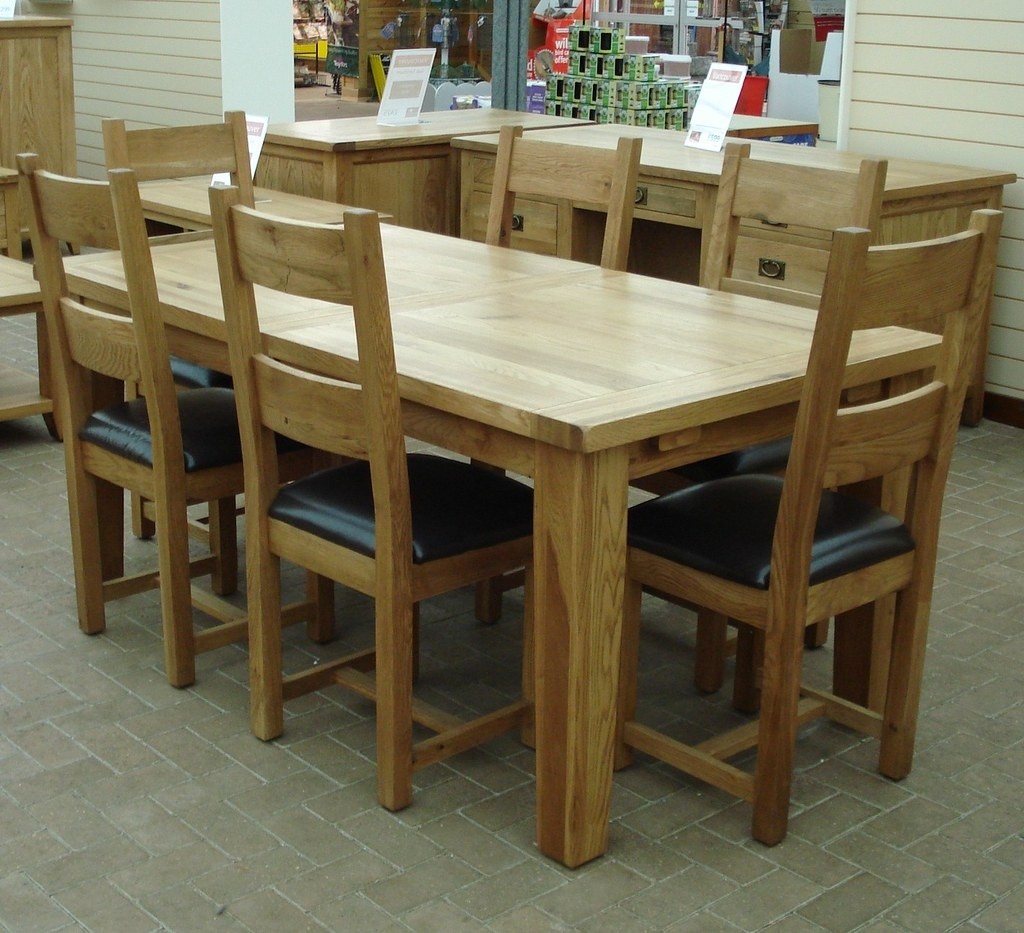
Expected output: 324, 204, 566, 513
544, 25, 702, 131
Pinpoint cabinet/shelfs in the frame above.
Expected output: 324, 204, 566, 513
252, 108, 597, 239
0, 16, 81, 254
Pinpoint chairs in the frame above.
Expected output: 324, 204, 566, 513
20, 113, 1005, 867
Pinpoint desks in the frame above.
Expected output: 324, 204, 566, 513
134, 181, 393, 245
0, 254, 57, 444
35, 224, 943, 869
0, 167, 21, 259
451, 124, 1016, 428
688, 108, 818, 150
